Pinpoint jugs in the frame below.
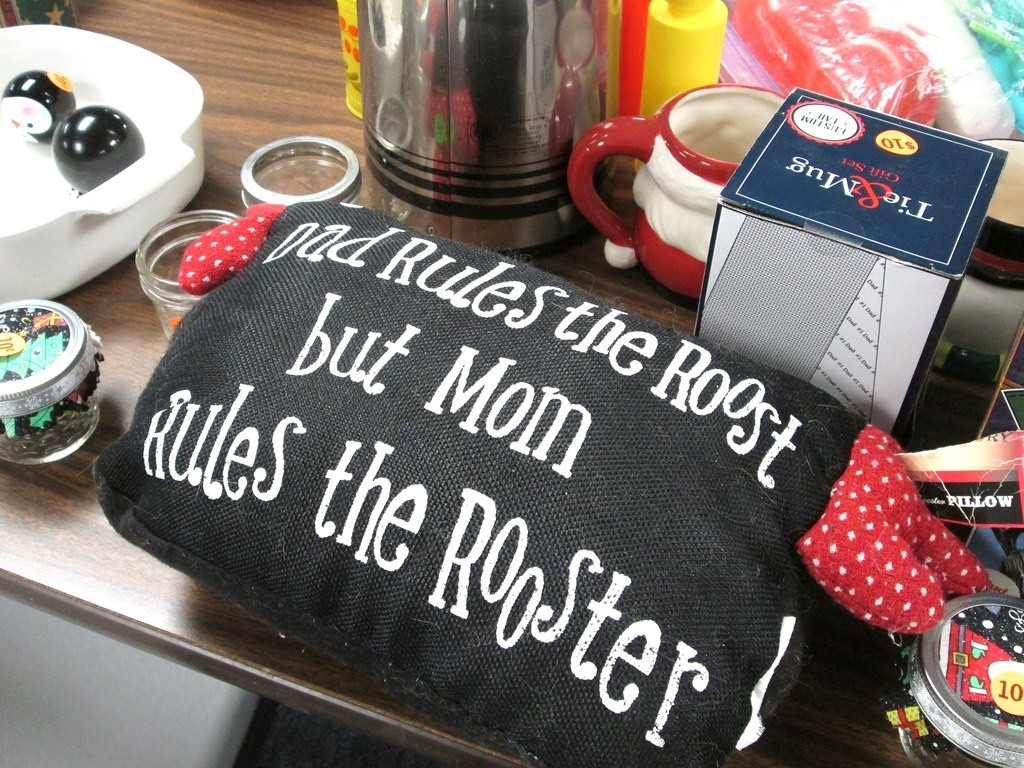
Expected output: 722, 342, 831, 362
357, 0, 621, 252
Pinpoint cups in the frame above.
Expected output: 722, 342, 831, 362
337, 0, 363, 120
942, 138, 1024, 354
568, 84, 788, 299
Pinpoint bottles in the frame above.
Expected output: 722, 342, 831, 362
621, 0, 728, 171
0, 300, 100, 465
896, 591, 1024, 768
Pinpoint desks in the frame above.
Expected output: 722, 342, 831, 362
0, 0, 1011, 768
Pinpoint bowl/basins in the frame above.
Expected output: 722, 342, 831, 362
0, 25, 205, 306
136, 209, 242, 341
241, 135, 362, 209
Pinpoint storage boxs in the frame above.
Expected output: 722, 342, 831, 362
693, 86, 1009, 432
